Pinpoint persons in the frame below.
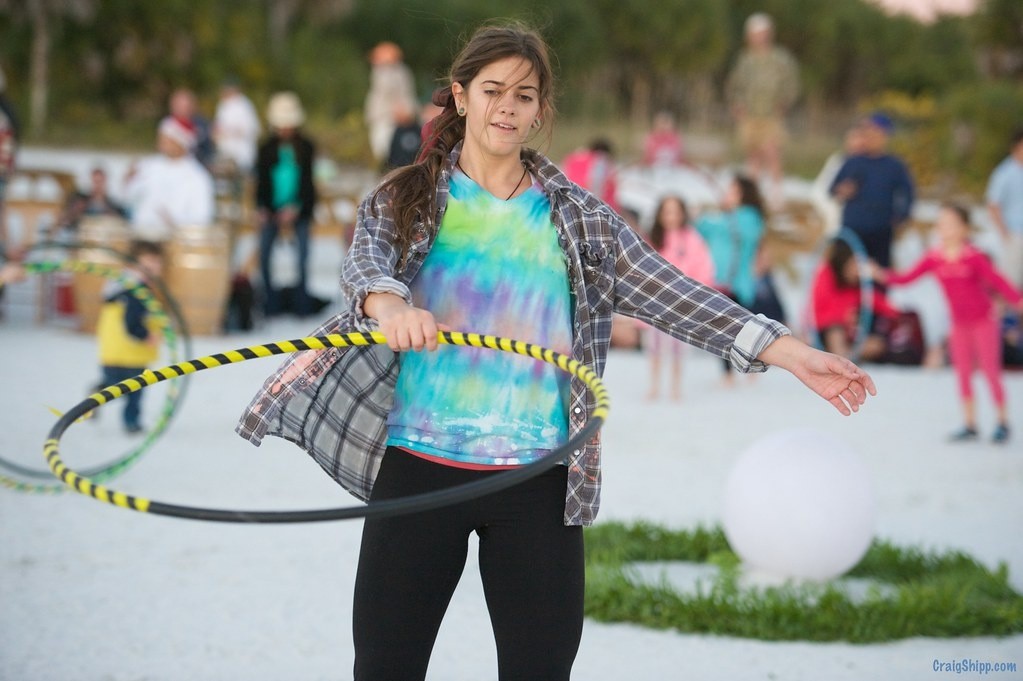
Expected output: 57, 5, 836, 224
235, 17, 876, 681
360, 38, 419, 183
641, 110, 684, 165
50, 81, 329, 325
687, 115, 1023, 368
863, 204, 1023, 445
0, 71, 18, 317
88, 241, 172, 434
636, 197, 714, 402
720, 12, 802, 178
563, 134, 623, 209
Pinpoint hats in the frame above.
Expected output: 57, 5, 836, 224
160, 116, 198, 149
873, 109, 894, 133
266, 91, 307, 128
377, 42, 402, 65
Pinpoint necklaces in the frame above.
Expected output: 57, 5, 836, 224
456, 162, 528, 200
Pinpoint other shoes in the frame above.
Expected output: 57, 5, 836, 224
949, 425, 977, 440
124, 422, 142, 434
992, 425, 1011, 443
